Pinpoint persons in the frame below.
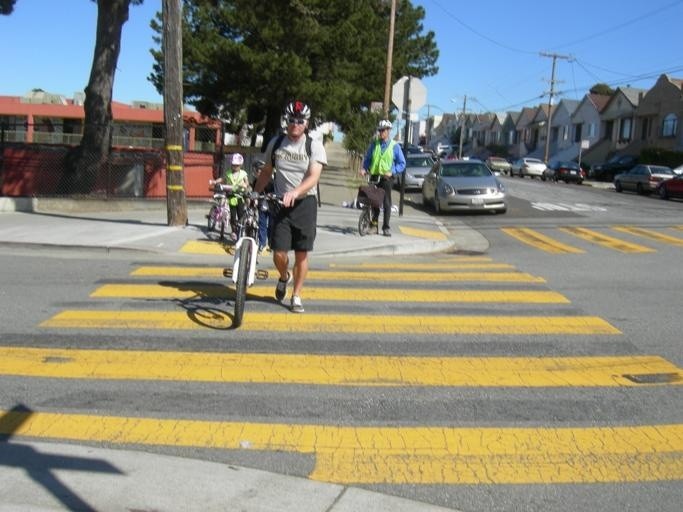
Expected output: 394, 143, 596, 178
248, 160, 274, 254
360, 119, 406, 238
252, 101, 329, 314
209, 152, 250, 242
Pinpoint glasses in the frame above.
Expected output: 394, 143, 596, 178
379, 129, 384, 132
286, 117, 307, 126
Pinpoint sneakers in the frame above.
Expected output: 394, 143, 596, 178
383, 228, 391, 236
230, 232, 237, 240
259, 245, 264, 251
290, 296, 305, 313
367, 225, 378, 234
276, 271, 293, 302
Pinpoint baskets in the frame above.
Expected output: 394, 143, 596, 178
357, 184, 385, 209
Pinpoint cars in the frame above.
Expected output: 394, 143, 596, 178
542, 161, 584, 184
400, 138, 469, 160
397, 154, 436, 190
485, 157, 513, 176
511, 158, 547, 178
614, 165, 675, 193
420, 160, 508, 215
658, 174, 682, 199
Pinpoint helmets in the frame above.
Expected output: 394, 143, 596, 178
230, 153, 244, 165
378, 120, 392, 130
250, 159, 266, 179
285, 101, 311, 119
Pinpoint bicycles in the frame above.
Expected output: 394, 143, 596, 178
205, 179, 230, 239
357, 172, 394, 235
221, 185, 281, 327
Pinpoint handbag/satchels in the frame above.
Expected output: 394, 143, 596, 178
359, 186, 385, 207
